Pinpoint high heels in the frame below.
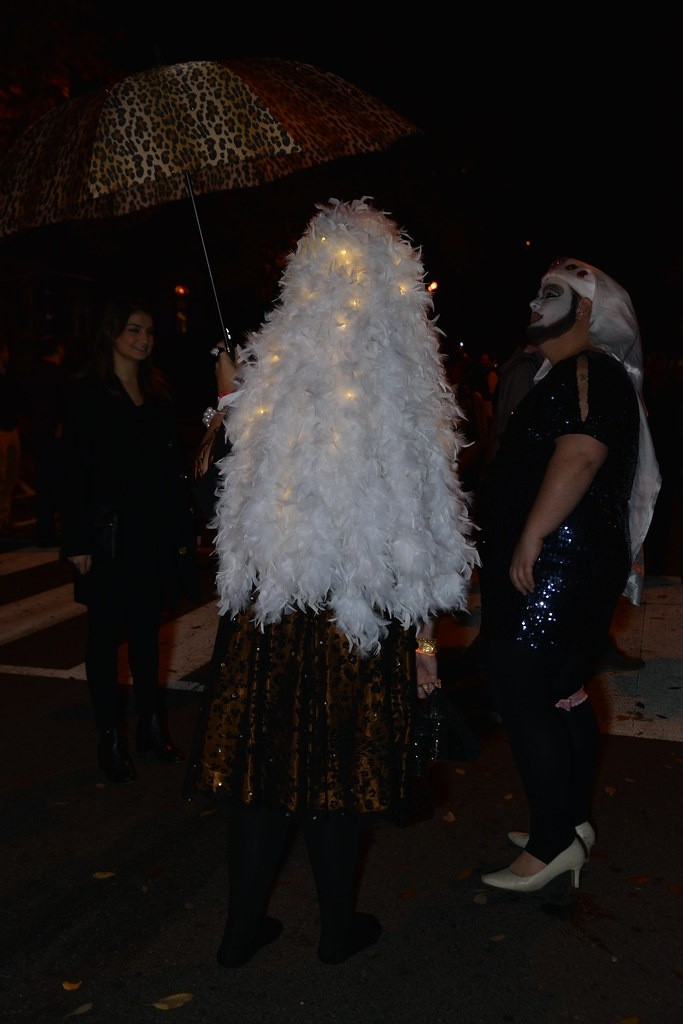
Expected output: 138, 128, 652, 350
507, 821, 596, 865
481, 837, 585, 893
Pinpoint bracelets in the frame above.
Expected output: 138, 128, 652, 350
218, 387, 237, 398
415, 637, 438, 657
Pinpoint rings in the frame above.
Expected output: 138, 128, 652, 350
79, 560, 85, 565
71, 561, 78, 567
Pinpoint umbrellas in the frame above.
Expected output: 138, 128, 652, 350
0, 58, 422, 355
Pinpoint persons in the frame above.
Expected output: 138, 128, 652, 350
55, 296, 190, 784
0, 340, 21, 532
192, 195, 481, 968
474, 255, 634, 891
441, 351, 498, 458
32, 332, 93, 548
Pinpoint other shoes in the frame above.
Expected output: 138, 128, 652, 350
318, 911, 382, 966
96, 736, 138, 786
216, 915, 283, 969
134, 710, 164, 752
